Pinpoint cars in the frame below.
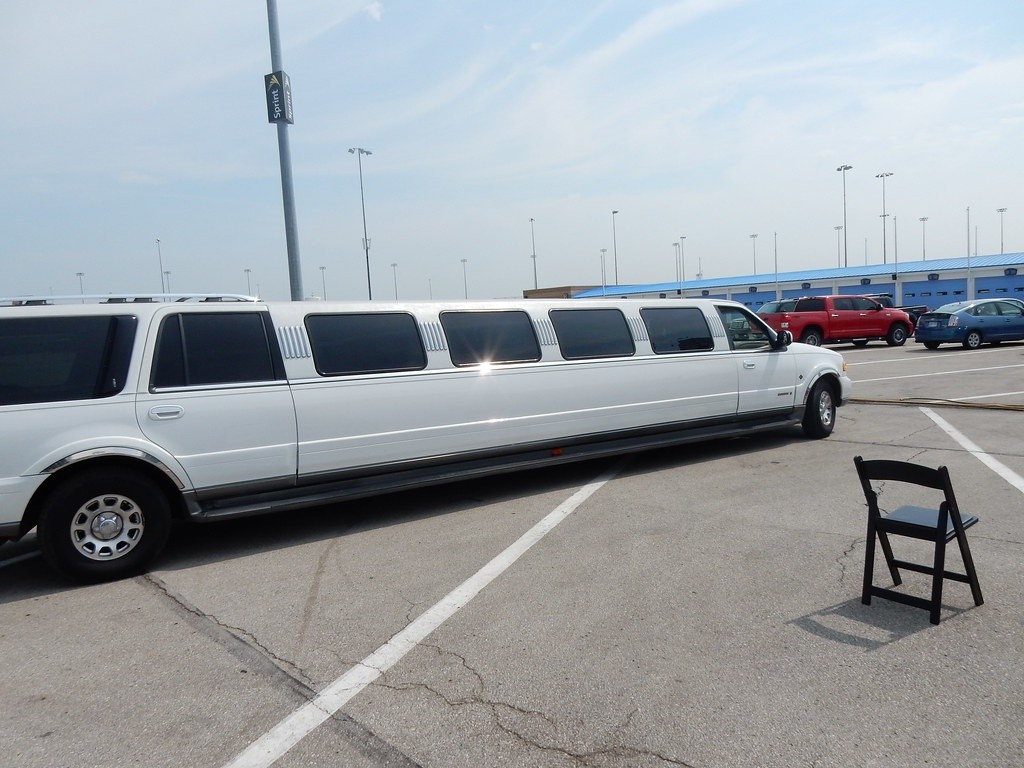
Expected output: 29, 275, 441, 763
914, 298, 1024, 351
0, 291, 852, 591
728, 316, 763, 340
992, 297, 1024, 316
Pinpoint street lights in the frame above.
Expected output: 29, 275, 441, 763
391, 263, 398, 300
834, 226, 843, 268
460, 259, 468, 301
995, 208, 1007, 255
612, 209, 618, 286
244, 268, 251, 297
680, 236, 687, 282
919, 217, 928, 262
163, 271, 172, 302
156, 238, 165, 303
320, 266, 327, 301
600, 247, 608, 288
672, 242, 679, 283
750, 234, 758, 275
348, 146, 373, 301
76, 272, 85, 304
836, 164, 853, 268
874, 171, 893, 265
529, 217, 536, 290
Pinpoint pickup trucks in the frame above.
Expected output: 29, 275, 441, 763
749, 294, 914, 347
863, 293, 929, 321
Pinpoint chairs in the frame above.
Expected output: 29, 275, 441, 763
853, 455, 984, 625
